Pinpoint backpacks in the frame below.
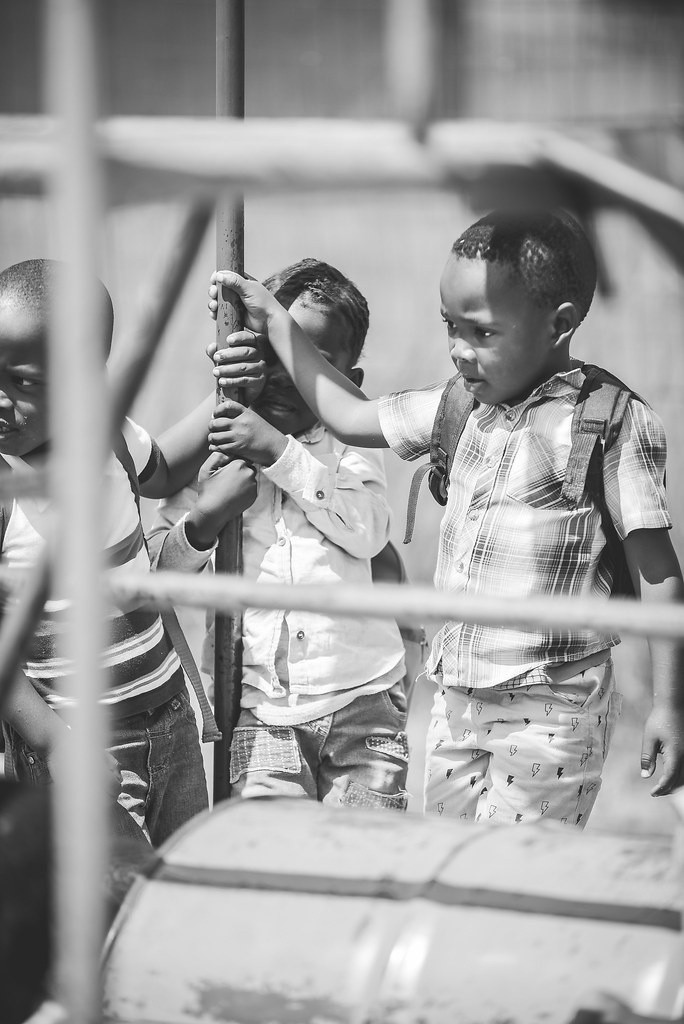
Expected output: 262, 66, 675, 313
371, 540, 428, 712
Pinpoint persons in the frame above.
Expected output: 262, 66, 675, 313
0, 256, 268, 852
0, 631, 125, 1024
136, 254, 410, 813
205, 197, 683, 841
